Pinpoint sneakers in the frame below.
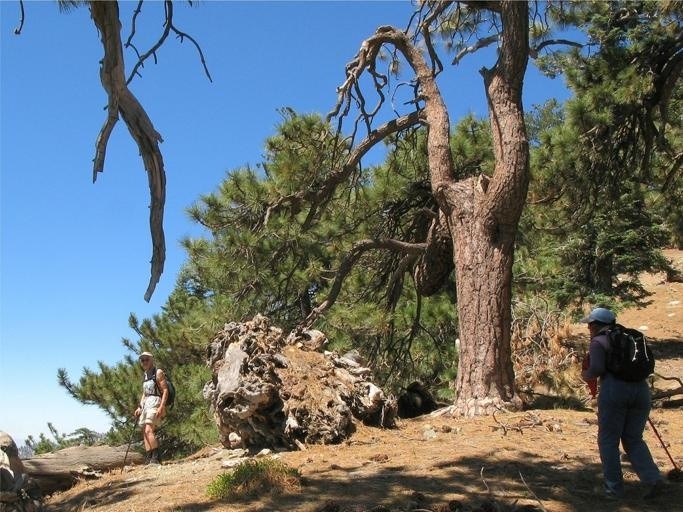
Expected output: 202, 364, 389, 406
144, 456, 161, 464
643, 483, 669, 501
595, 481, 620, 500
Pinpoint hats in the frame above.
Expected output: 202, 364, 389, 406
580, 308, 616, 324
139, 352, 153, 359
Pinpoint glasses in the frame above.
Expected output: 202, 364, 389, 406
141, 358, 149, 361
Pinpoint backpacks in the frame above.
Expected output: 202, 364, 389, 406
152, 368, 175, 405
596, 325, 654, 382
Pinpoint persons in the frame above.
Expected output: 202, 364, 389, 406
580, 306, 664, 502
134, 351, 169, 465
